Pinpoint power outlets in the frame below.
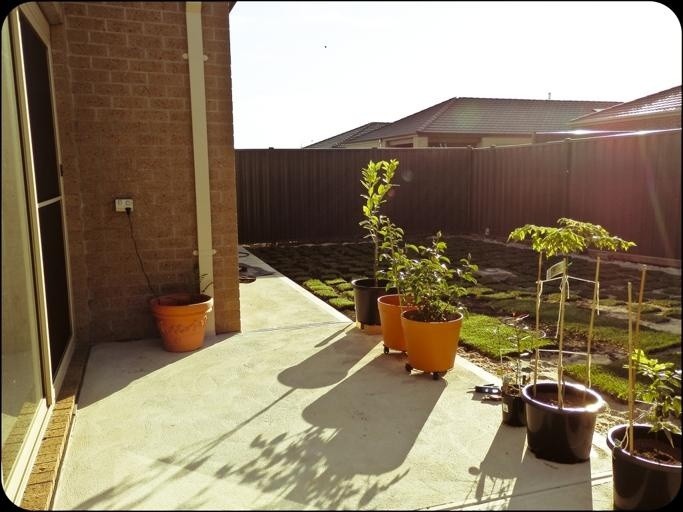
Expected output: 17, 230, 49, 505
114, 198, 133, 212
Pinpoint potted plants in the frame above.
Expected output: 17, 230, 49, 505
501, 312, 535, 428
507, 218, 637, 463
350, 159, 401, 330
400, 231, 478, 379
143, 270, 214, 353
606, 348, 682, 512
377, 216, 427, 356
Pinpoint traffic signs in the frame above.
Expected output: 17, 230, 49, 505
114, 198, 133, 212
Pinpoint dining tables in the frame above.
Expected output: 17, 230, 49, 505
143, 270, 214, 353
